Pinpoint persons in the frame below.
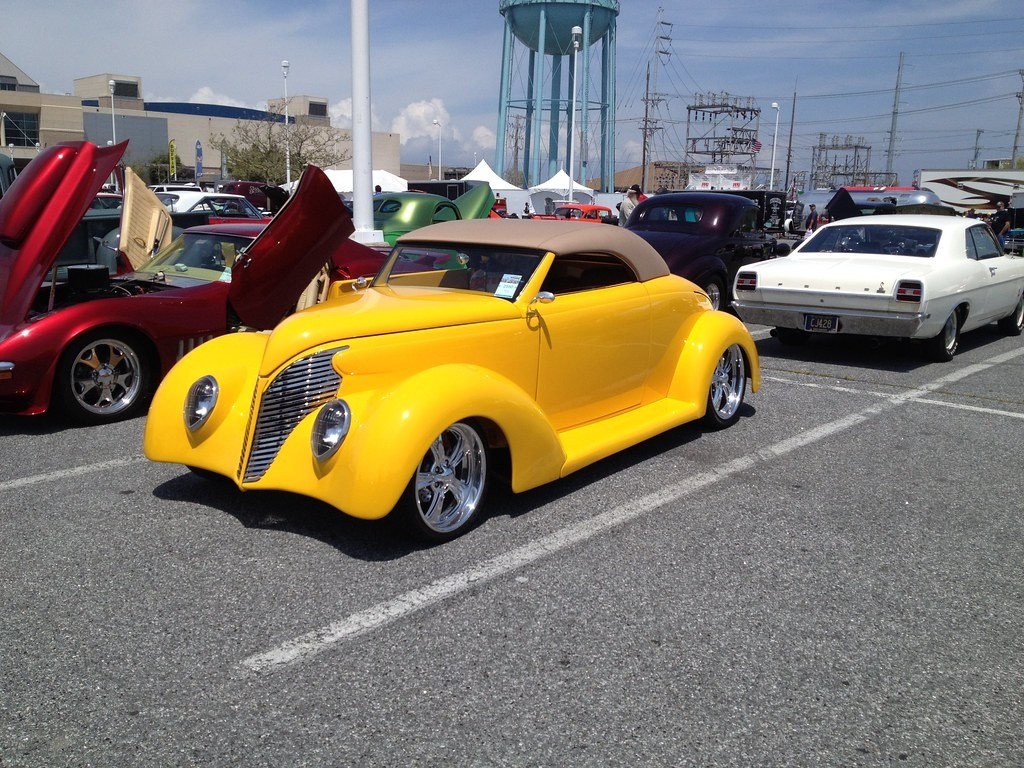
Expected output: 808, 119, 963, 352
619, 183, 648, 226
523, 202, 529, 213
373, 185, 383, 198
964, 201, 1010, 253
806, 204, 818, 235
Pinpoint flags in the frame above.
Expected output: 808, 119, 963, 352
752, 138, 761, 153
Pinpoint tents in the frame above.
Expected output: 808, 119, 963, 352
460, 158, 522, 191
528, 169, 594, 214
280, 169, 409, 194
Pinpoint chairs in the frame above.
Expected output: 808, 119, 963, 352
856, 242, 884, 254
539, 270, 576, 294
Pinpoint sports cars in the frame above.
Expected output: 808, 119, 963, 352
141, 217, 763, 543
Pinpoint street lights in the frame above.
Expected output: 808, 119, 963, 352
432, 119, 444, 182
769, 101, 782, 189
106, 79, 121, 192
278, 61, 293, 196
567, 25, 584, 205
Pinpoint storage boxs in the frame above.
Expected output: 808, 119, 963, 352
67, 264, 111, 292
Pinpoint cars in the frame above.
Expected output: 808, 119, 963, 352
726, 213, 1024, 363
0, 136, 1024, 420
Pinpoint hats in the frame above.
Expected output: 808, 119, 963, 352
630, 184, 642, 195
995, 201, 1005, 207
809, 204, 816, 207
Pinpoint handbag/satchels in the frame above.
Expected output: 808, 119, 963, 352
803, 228, 813, 240
